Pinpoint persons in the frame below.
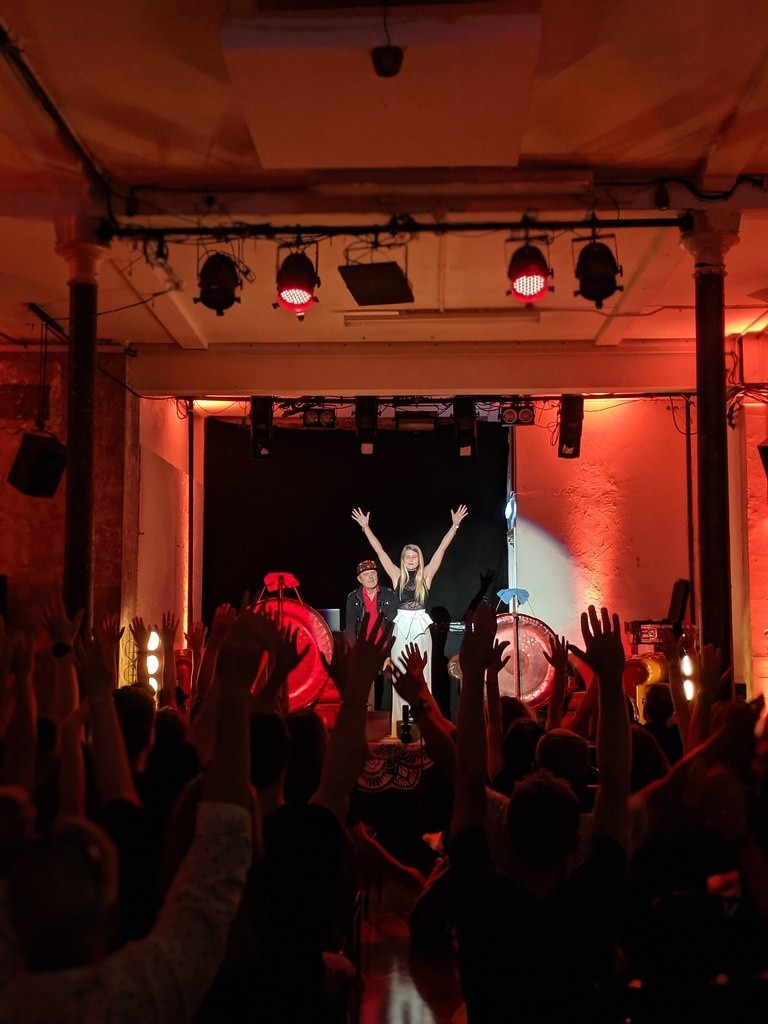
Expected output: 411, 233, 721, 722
351, 505, 469, 734
1, 572, 768, 1024
342, 558, 398, 703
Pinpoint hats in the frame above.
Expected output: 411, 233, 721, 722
357, 560, 377, 575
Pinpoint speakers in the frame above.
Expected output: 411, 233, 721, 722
8, 434, 68, 500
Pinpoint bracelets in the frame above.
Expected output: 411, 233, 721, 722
361, 524, 368, 531
452, 524, 460, 530
